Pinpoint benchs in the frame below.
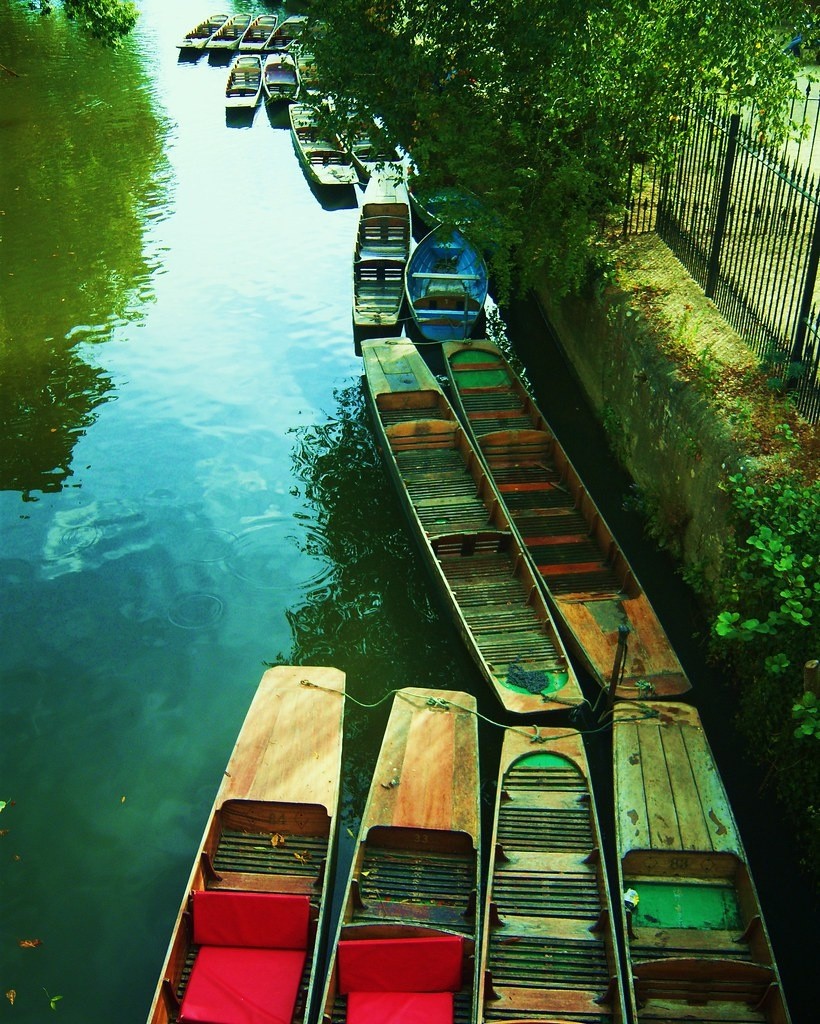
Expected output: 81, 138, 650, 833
177, 890, 309, 1024
338, 937, 465, 1024
412, 272, 481, 280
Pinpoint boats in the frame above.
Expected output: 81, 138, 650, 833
403, 220, 490, 342
476, 724, 631, 1024
288, 102, 360, 185
405, 165, 517, 243
147, 664, 347, 1024
351, 163, 413, 330
327, 97, 403, 180
611, 701, 795, 1024
357, 336, 587, 718
175, 12, 343, 109
315, 686, 484, 1023
435, 338, 693, 701
